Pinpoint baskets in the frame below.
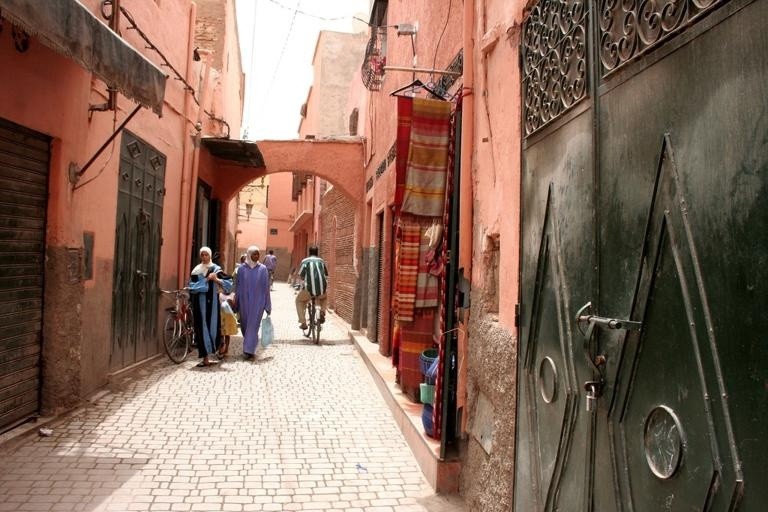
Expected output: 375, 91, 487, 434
419, 348, 438, 375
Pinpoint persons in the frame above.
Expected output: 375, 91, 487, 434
188, 246, 278, 366
296, 246, 328, 329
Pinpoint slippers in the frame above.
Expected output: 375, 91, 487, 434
219, 352, 225, 360
197, 361, 210, 367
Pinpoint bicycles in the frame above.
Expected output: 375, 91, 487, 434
294, 281, 325, 344
269, 269, 275, 288
159, 286, 197, 365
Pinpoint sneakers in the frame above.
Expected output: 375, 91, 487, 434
320, 312, 324, 322
300, 325, 308, 329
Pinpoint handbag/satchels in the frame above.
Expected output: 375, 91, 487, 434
262, 317, 273, 346
